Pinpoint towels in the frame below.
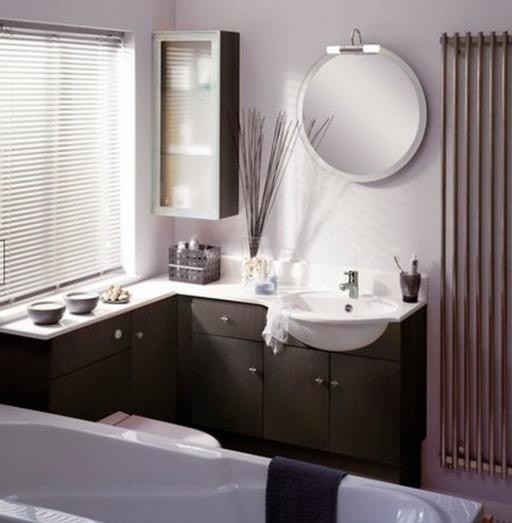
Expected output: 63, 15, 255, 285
260, 293, 311, 354
265, 456, 348, 522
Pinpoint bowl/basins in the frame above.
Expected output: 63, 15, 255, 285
64, 291, 101, 314
27, 300, 66, 325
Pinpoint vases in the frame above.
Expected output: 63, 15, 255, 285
240, 235, 265, 280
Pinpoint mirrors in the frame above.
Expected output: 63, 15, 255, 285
296, 43, 428, 184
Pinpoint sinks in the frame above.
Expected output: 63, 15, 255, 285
279, 289, 398, 355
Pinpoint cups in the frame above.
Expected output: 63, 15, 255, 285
399, 272, 421, 303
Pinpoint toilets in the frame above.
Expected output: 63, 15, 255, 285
92, 409, 223, 449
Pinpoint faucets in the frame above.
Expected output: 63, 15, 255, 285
339, 271, 360, 300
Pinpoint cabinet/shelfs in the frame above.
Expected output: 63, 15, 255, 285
129, 295, 177, 423
150, 30, 240, 221
263, 304, 427, 489
177, 295, 263, 438
0, 312, 129, 422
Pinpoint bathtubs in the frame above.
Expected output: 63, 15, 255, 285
0, 404, 436, 523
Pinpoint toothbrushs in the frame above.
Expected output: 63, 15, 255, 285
393, 256, 404, 275
